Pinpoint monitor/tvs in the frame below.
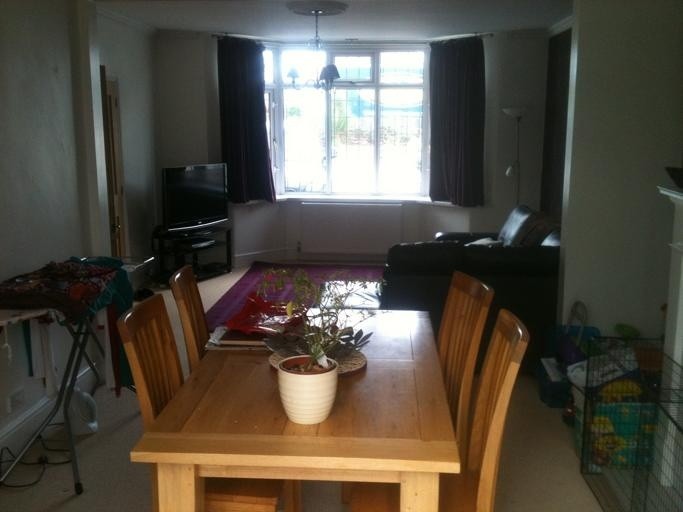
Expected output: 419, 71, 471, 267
162, 162, 229, 232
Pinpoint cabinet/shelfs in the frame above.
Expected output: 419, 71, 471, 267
149, 218, 234, 288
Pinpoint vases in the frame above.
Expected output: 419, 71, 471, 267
273, 354, 342, 426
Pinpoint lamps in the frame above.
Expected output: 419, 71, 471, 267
278, 0, 350, 91
499, 105, 527, 208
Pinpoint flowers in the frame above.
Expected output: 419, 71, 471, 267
248, 254, 394, 371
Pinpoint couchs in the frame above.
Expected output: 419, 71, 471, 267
378, 200, 564, 381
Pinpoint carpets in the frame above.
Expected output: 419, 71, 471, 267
191, 258, 388, 342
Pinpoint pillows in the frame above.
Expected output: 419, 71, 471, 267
463, 232, 505, 251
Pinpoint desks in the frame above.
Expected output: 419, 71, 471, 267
127, 306, 463, 512
0, 254, 158, 496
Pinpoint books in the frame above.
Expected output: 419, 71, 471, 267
204, 325, 273, 350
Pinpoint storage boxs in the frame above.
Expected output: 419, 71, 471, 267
563, 369, 658, 478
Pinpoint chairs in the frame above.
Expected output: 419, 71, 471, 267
114, 288, 298, 511
166, 264, 303, 512
337, 305, 533, 510
338, 268, 497, 511
